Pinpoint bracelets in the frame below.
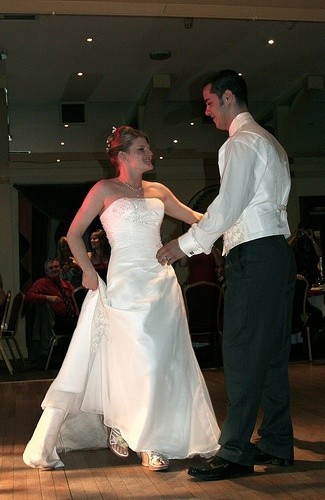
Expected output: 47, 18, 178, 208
313, 240, 317, 244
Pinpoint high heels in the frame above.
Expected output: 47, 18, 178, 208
141, 448, 169, 470
107, 427, 129, 458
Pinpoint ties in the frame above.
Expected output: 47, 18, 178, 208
53, 279, 72, 311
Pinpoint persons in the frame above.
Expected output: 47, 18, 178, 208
52, 235, 88, 290
79, 228, 111, 281
286, 222, 323, 288
67, 125, 207, 472
24, 259, 83, 339
152, 69, 294, 483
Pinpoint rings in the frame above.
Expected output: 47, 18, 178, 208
165, 256, 169, 260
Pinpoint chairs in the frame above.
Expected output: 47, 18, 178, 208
292, 277, 312, 362
184, 280, 222, 366
41, 299, 71, 371
2, 292, 30, 373
72, 286, 88, 314
0, 290, 15, 377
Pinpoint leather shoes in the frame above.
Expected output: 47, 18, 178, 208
188, 456, 253, 478
250, 442, 294, 466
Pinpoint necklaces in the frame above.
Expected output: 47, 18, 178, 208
119, 175, 145, 197
